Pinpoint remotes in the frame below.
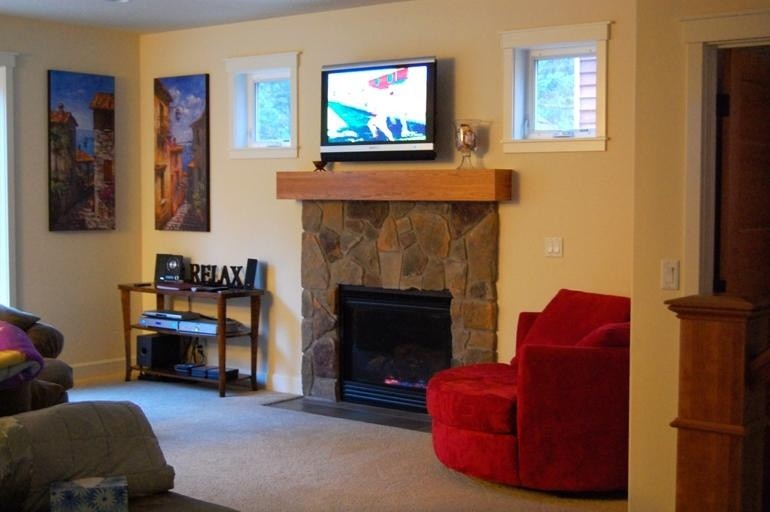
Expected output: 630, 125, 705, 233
134, 282, 152, 287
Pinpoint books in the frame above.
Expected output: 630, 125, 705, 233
172, 361, 240, 382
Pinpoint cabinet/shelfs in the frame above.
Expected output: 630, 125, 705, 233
117, 281, 264, 397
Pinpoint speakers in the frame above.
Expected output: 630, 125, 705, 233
244, 257, 257, 290
154, 253, 185, 284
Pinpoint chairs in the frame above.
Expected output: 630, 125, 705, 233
425, 279, 629, 494
0, 305, 177, 510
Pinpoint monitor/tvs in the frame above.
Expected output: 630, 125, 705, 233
319, 55, 438, 163
